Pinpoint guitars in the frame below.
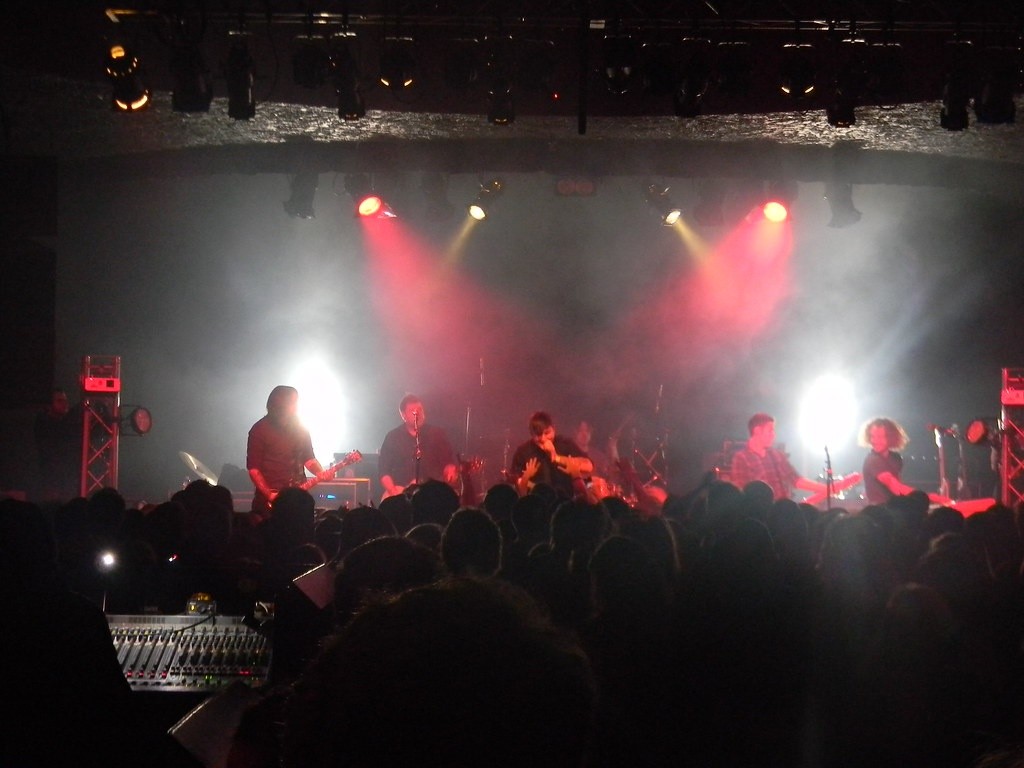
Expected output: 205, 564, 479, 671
381, 477, 425, 503
807, 474, 861, 507
253, 450, 364, 517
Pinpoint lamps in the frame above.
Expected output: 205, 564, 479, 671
226, 59, 256, 120
674, 74, 710, 118
344, 173, 385, 218
489, 78, 516, 124
693, 178, 725, 228
287, 171, 321, 220
555, 175, 598, 195
823, 179, 861, 230
291, 47, 333, 89
377, 47, 415, 89
967, 417, 1001, 451
116, 404, 153, 437
939, 76, 1014, 131
638, 179, 683, 225
102, 37, 152, 112
170, 62, 215, 113
469, 177, 504, 220
762, 182, 801, 221
826, 73, 856, 128
333, 67, 366, 120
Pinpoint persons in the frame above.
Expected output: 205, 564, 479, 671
511, 413, 592, 496
378, 394, 458, 495
865, 420, 954, 506
1, 455, 1024, 767
730, 415, 838, 500
247, 385, 335, 510
562, 418, 603, 465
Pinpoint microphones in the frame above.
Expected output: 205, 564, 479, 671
480, 358, 485, 386
928, 424, 954, 434
411, 410, 419, 416
655, 385, 662, 411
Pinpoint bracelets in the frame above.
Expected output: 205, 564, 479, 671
556, 456, 560, 462
